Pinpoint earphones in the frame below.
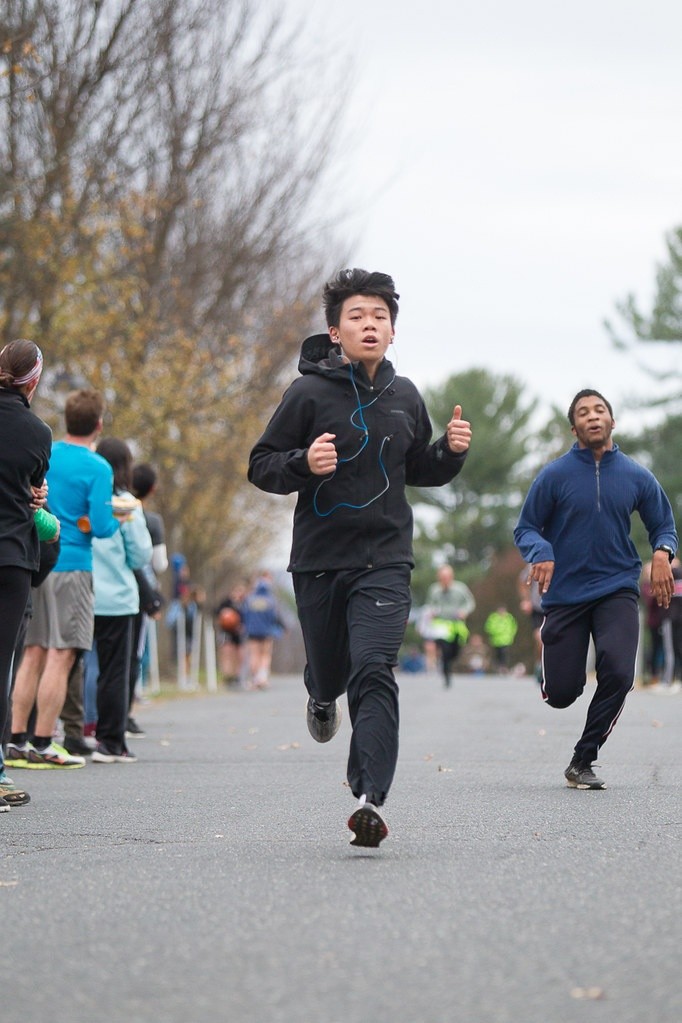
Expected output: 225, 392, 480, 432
333, 334, 341, 340
389, 335, 395, 344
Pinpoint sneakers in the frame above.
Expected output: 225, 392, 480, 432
347, 798, 389, 849
25, 739, 86, 770
306, 695, 343, 744
3, 739, 36, 768
90, 740, 139, 763
563, 760, 608, 790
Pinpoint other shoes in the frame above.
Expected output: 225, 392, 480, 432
0, 788, 30, 803
0, 776, 14, 788
63, 742, 96, 756
124, 717, 147, 739
82, 723, 100, 746
0, 798, 11, 811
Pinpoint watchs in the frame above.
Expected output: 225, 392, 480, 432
654, 544, 674, 562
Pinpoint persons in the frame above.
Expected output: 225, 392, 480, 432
514, 389, 679, 790
644, 558, 682, 689
426, 564, 476, 682
398, 636, 487, 673
216, 581, 285, 689
0, 338, 169, 813
485, 605, 517, 674
246, 269, 472, 849
